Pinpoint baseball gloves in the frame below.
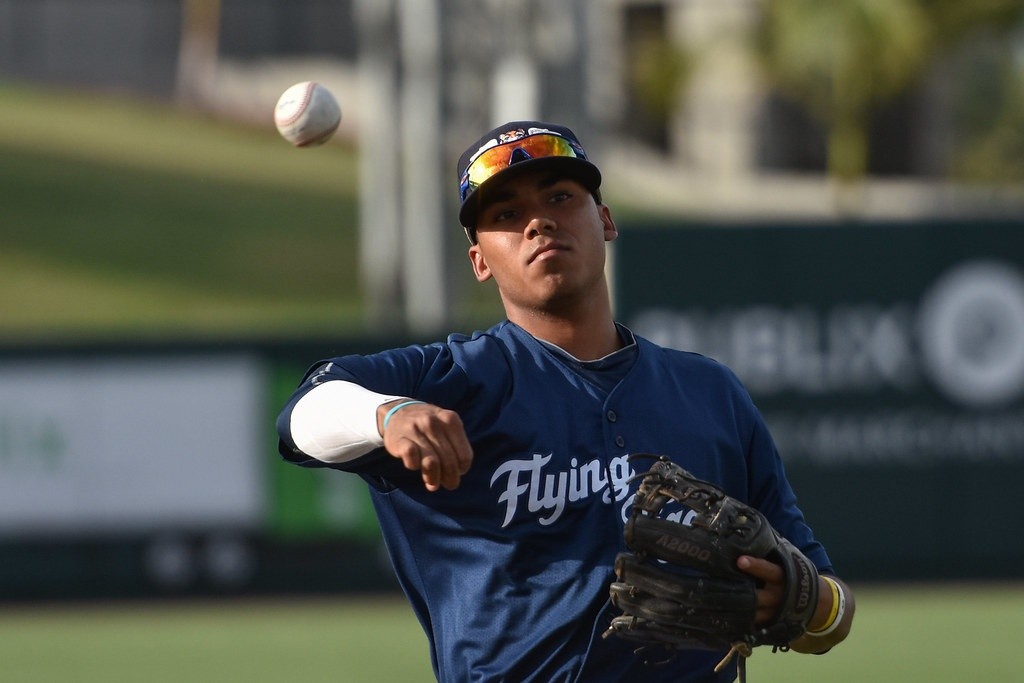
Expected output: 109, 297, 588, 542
601, 452, 826, 669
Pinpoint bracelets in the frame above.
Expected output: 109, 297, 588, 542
383, 401, 425, 428
806, 575, 846, 638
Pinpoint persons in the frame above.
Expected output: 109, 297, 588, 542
275, 122, 855, 683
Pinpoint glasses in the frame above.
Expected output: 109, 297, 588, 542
458, 134, 589, 205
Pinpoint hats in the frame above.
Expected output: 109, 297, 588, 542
457, 121, 602, 245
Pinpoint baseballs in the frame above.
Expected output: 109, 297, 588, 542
272, 77, 345, 153
